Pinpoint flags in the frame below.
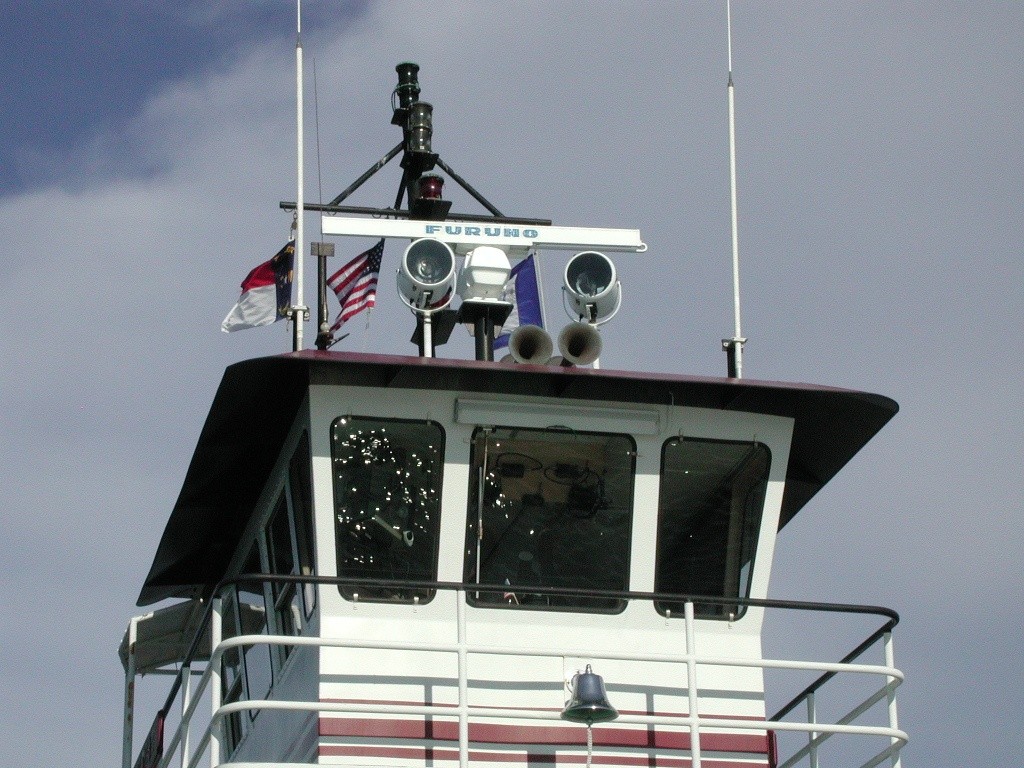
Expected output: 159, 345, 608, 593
493, 254, 545, 350
221, 240, 295, 333
326, 238, 386, 334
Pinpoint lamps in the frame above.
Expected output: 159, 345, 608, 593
561, 250, 622, 369
396, 237, 457, 357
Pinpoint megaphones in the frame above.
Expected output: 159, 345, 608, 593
556, 321, 603, 367
508, 324, 554, 366
